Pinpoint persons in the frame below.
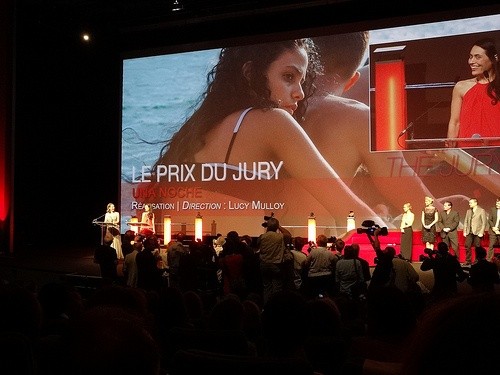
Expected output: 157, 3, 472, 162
263, 32, 470, 248
105, 203, 124, 259
420, 242, 464, 300
365, 228, 396, 301
422, 196, 438, 251
94, 234, 117, 278
305, 235, 336, 298
0, 231, 500, 375
133, 38, 387, 241
437, 201, 460, 259
400, 202, 415, 260
487, 198, 500, 262
257, 218, 293, 263
140, 204, 155, 235
463, 199, 487, 265
445, 40, 500, 150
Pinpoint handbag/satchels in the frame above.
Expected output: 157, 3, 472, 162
355, 280, 366, 294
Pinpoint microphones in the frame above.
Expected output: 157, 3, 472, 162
397, 76, 460, 149
484, 70, 494, 87
